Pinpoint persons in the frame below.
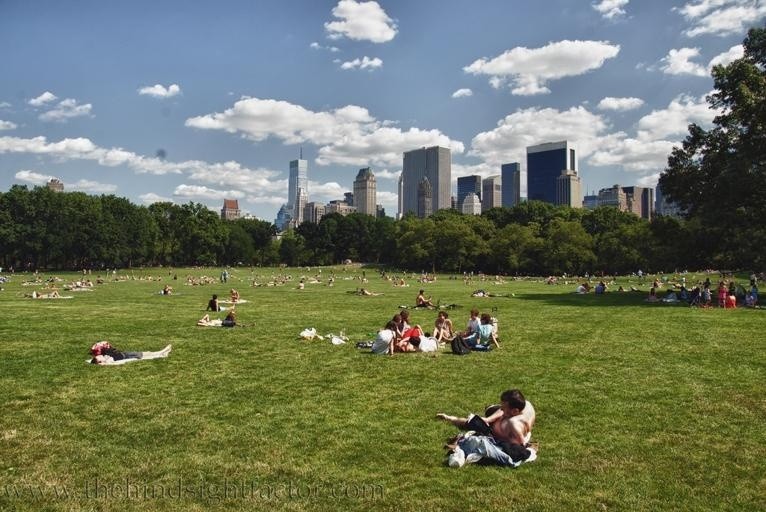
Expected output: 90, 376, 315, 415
0, 257, 766, 355
436, 390, 535, 445
89, 345, 171, 365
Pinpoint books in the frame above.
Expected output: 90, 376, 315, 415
467, 412, 491, 431
